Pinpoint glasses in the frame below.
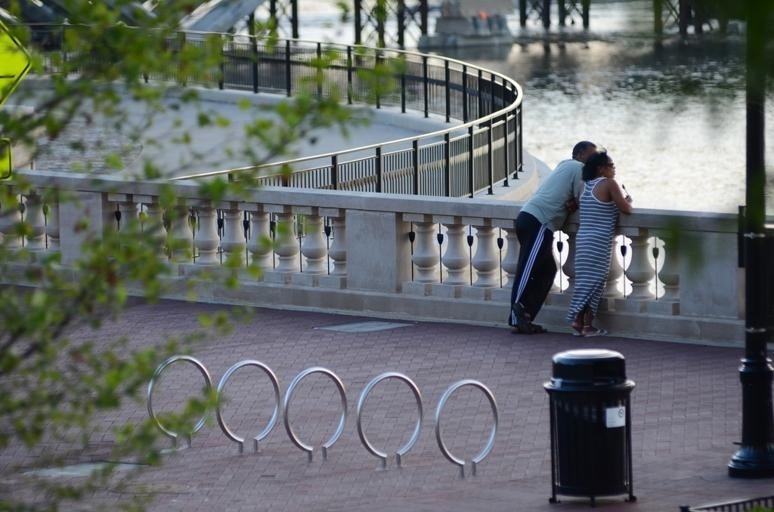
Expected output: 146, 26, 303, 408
606, 163, 614, 168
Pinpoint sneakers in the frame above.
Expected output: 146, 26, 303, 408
509, 302, 545, 334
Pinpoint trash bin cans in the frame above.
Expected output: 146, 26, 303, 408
543, 348, 637, 506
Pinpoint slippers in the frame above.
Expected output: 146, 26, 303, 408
571, 320, 607, 338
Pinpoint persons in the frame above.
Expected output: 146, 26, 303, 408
567, 150, 633, 338
507, 140, 596, 335
